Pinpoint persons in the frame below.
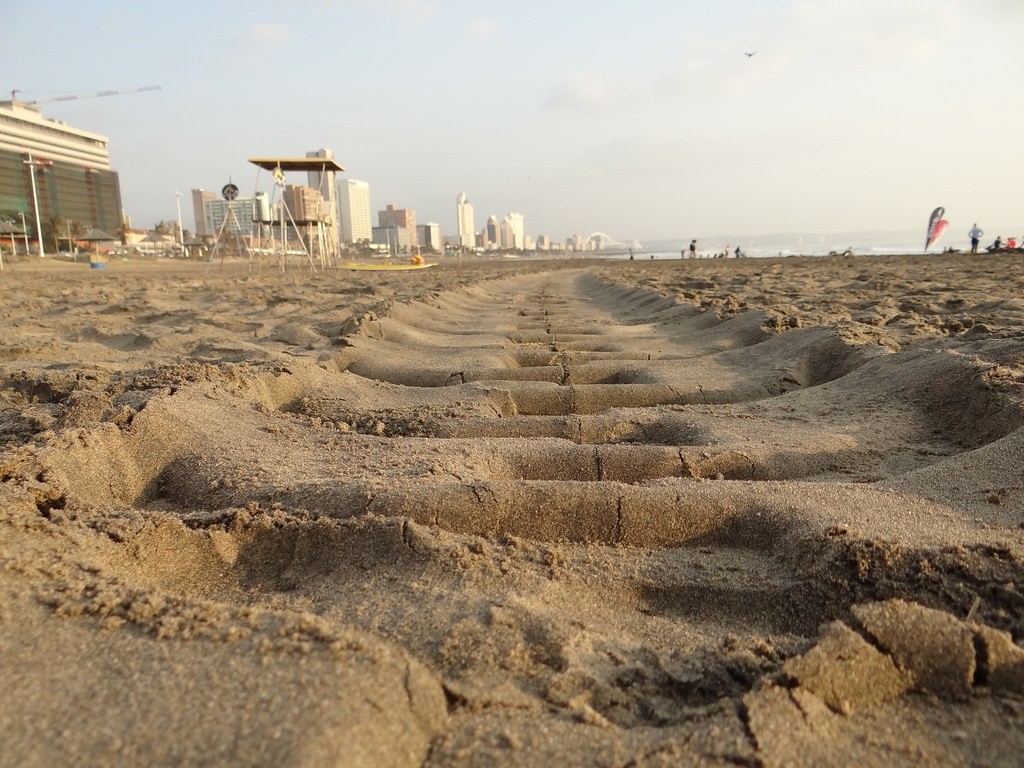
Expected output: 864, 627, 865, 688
968, 223, 983, 253
690, 239, 696, 257
629, 249, 633, 260
725, 246, 740, 258
944, 246, 953, 254
995, 237, 1015, 248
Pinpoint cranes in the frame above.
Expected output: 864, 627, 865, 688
11, 85, 161, 104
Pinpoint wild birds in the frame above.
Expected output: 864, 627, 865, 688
744, 51, 756, 58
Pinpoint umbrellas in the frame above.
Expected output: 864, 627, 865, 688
211, 242, 232, 255
182, 237, 205, 258
140, 233, 169, 261
76, 227, 116, 261
0, 220, 25, 269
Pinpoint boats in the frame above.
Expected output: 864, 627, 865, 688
337, 263, 439, 270
